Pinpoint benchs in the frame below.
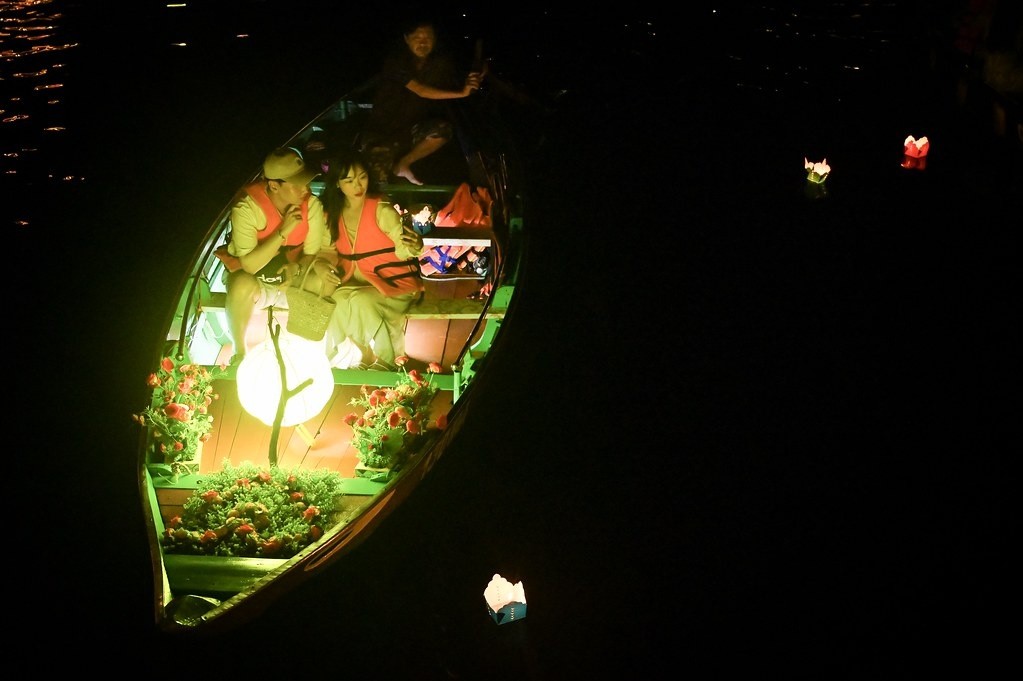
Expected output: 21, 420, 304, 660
422, 227, 495, 246
190, 291, 494, 321
309, 181, 483, 196
200, 365, 454, 393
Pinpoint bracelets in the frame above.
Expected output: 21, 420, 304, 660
278, 230, 285, 241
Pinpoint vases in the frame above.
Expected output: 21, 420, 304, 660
353, 450, 413, 481
145, 421, 206, 479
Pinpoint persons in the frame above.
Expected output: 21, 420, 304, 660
221, 146, 425, 374
349, 12, 488, 188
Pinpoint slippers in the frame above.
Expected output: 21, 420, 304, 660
368, 362, 397, 371
351, 359, 379, 371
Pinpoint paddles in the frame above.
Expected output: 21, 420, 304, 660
473, 37, 536, 108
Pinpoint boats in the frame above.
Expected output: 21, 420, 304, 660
137, 49, 531, 640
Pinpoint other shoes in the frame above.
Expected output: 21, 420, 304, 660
229, 354, 244, 366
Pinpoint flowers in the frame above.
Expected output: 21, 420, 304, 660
130, 342, 229, 459
342, 353, 446, 452
164, 459, 342, 561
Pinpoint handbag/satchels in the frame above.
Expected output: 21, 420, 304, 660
286, 259, 337, 341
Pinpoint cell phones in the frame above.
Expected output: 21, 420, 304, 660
401, 212, 413, 242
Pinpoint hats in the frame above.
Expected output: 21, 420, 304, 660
262, 146, 322, 189
